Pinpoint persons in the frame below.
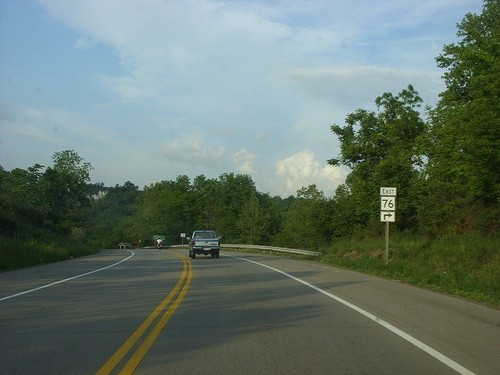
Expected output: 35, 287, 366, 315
195, 234, 202, 239
156, 237, 162, 248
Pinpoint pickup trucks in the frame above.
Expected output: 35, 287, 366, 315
187, 230, 220, 258
